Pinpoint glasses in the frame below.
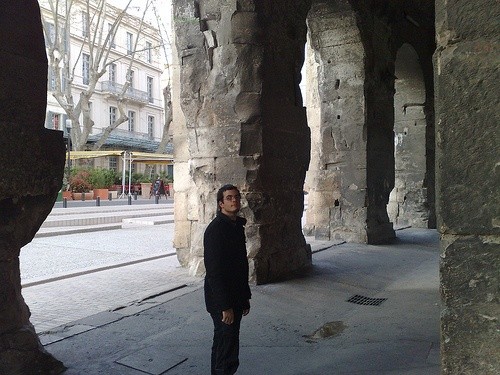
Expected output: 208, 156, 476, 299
221, 194, 241, 202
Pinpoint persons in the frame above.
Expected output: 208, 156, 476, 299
203, 184, 252, 375
149, 181, 169, 200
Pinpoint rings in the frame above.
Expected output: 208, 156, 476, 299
231, 322, 233, 323
